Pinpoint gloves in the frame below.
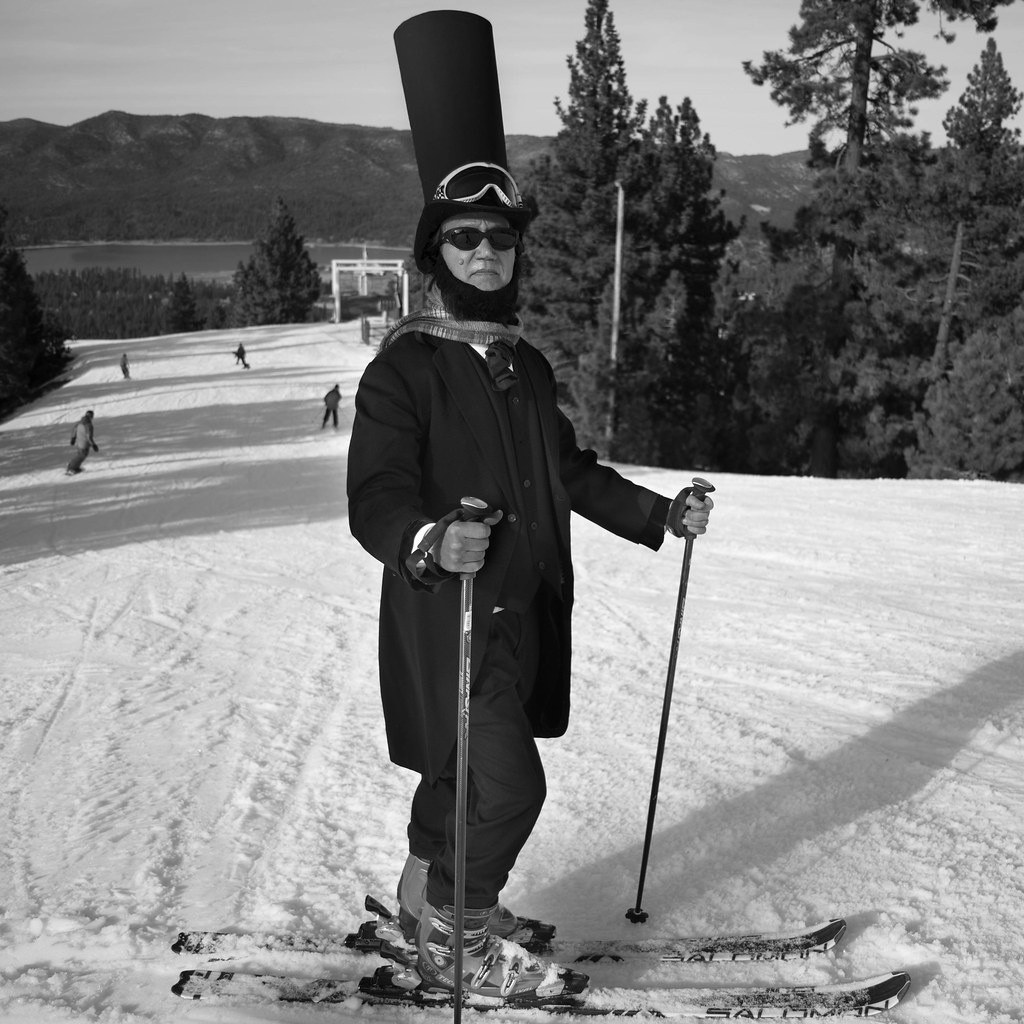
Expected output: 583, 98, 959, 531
93, 444, 99, 452
70, 437, 76, 445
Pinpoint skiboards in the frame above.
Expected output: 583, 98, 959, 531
171, 914, 912, 1018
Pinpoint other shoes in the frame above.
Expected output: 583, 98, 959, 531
415, 905, 547, 996
397, 851, 517, 944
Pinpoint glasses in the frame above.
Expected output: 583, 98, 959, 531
437, 226, 520, 251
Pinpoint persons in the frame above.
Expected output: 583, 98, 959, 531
66, 410, 98, 476
348, 160, 712, 1002
321, 384, 343, 428
121, 354, 131, 378
234, 344, 250, 368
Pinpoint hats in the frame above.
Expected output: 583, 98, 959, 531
393, 10, 539, 274
